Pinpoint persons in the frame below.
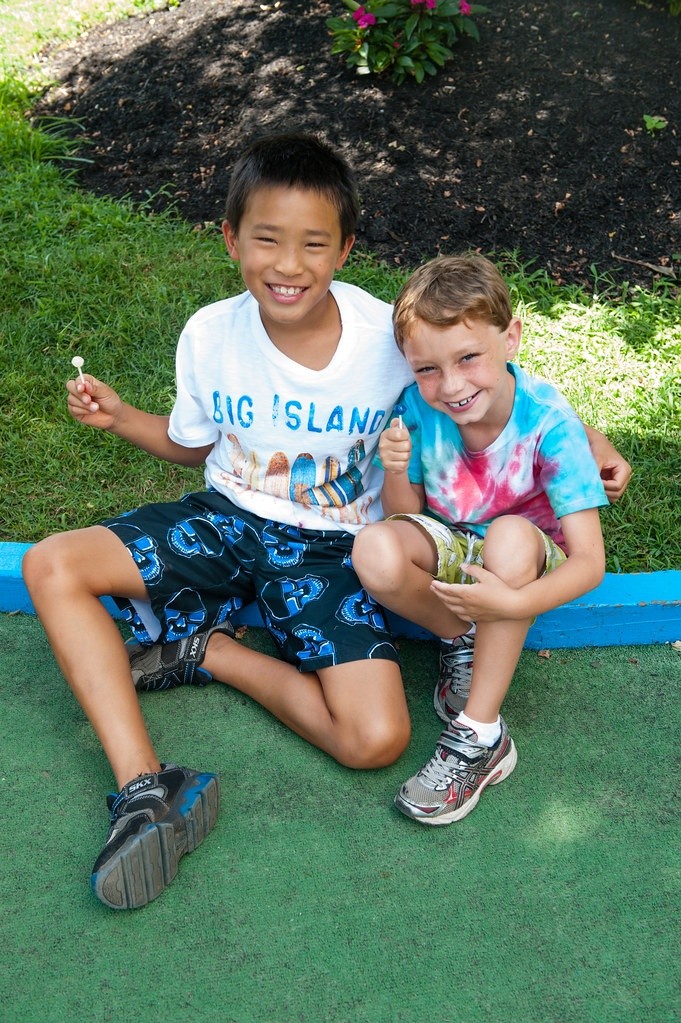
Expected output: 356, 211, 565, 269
350, 256, 611, 825
23, 134, 634, 911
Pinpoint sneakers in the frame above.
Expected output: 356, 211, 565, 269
393, 713, 518, 827
124, 617, 236, 692
433, 620, 478, 724
90, 763, 221, 910
